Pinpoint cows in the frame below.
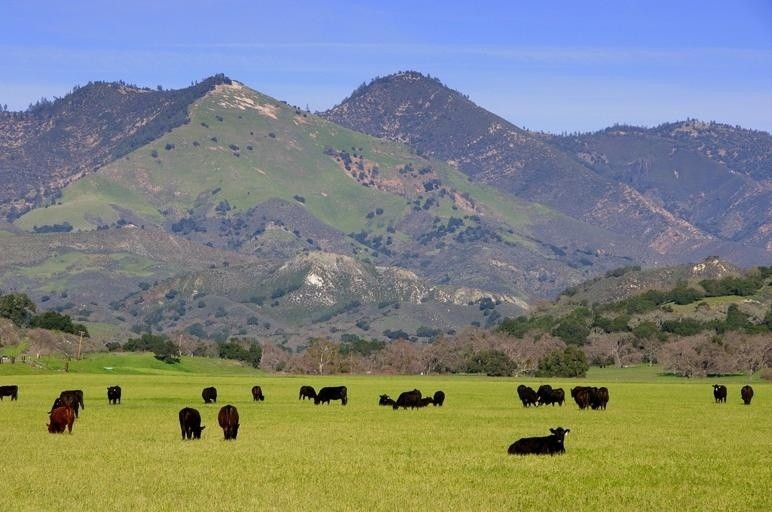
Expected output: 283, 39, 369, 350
218, 404, 240, 440
711, 384, 754, 405
202, 386, 217, 403
0, 386, 18, 401
179, 407, 205, 439
252, 386, 264, 401
379, 388, 445, 410
45, 390, 84, 434
107, 386, 121, 404
517, 384, 609, 410
298, 386, 347, 405
507, 427, 570, 456
